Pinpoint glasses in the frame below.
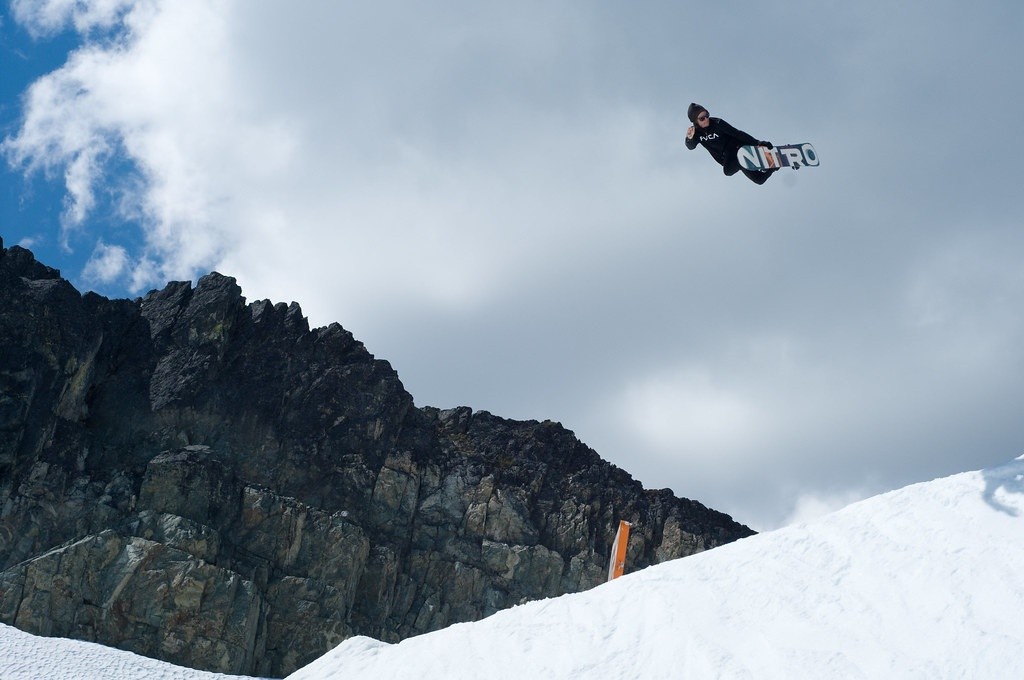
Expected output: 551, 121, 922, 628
697, 112, 709, 121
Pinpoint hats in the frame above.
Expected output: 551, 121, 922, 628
688, 103, 707, 122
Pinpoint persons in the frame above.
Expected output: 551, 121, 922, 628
685, 102, 800, 185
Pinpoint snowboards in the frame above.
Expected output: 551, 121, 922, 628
737, 142, 820, 171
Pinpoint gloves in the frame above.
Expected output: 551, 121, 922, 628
759, 141, 773, 150
